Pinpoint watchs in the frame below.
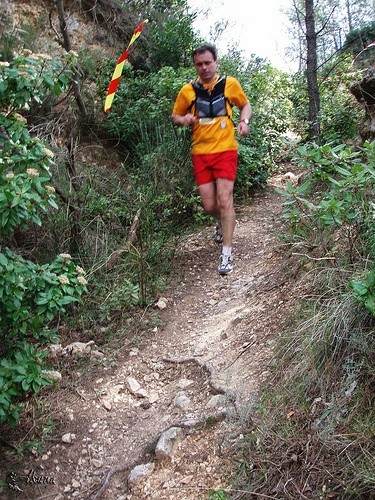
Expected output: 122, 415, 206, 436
239, 118, 249, 124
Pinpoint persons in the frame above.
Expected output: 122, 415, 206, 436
172, 44, 253, 276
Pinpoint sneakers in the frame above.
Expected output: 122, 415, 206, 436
218, 248, 234, 275
215, 224, 223, 244
